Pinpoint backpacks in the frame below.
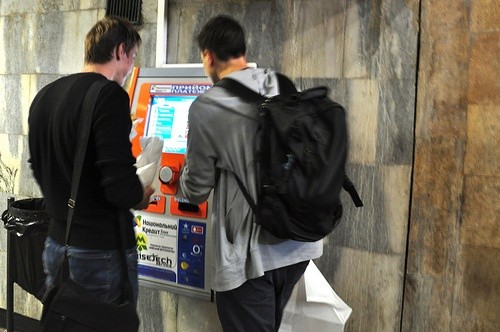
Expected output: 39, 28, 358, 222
213, 72, 349, 243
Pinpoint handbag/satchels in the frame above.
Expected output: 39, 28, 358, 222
39, 255, 140, 332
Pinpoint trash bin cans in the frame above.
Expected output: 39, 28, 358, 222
0, 197, 54, 302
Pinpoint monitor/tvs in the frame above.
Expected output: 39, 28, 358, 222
147, 96, 198, 154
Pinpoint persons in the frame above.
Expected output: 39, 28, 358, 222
27, 17, 153, 332
179, 13, 324, 332
